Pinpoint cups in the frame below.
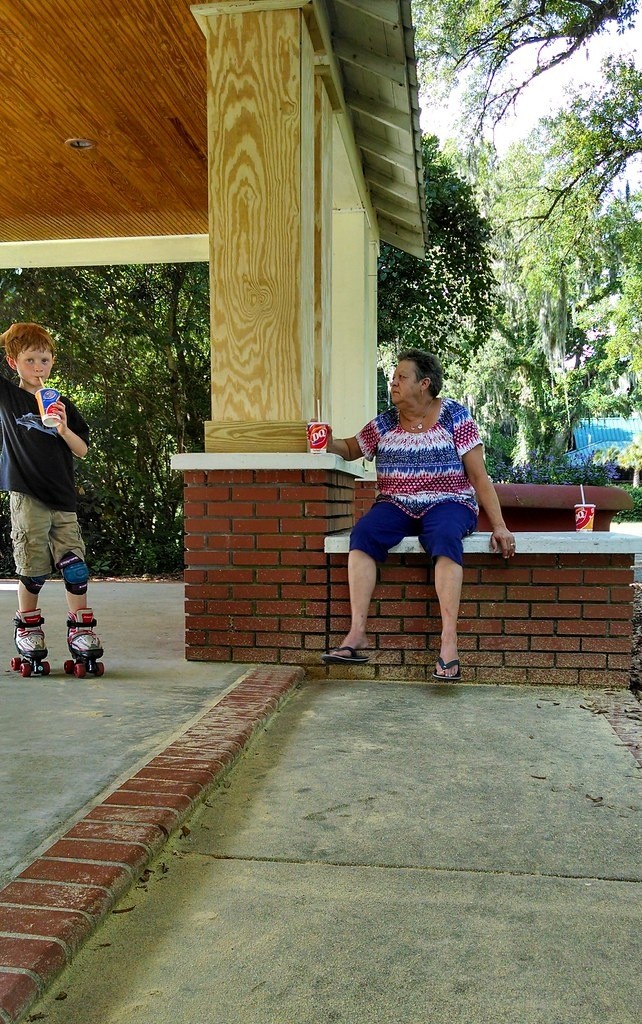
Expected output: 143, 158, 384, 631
306, 422, 328, 454
574, 504, 596, 533
34, 388, 62, 428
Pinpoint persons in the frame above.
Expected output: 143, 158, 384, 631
305, 349, 516, 681
0, 322, 104, 659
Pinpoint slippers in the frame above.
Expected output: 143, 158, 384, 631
432, 656, 462, 680
321, 646, 370, 663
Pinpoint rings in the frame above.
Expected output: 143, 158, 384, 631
510, 542, 515, 546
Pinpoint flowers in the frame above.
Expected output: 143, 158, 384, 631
480, 452, 622, 484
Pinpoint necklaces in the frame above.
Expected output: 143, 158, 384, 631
401, 415, 428, 431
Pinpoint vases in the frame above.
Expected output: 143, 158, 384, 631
473, 482, 634, 532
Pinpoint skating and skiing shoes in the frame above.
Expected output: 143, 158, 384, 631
64, 607, 104, 677
10, 608, 50, 677
